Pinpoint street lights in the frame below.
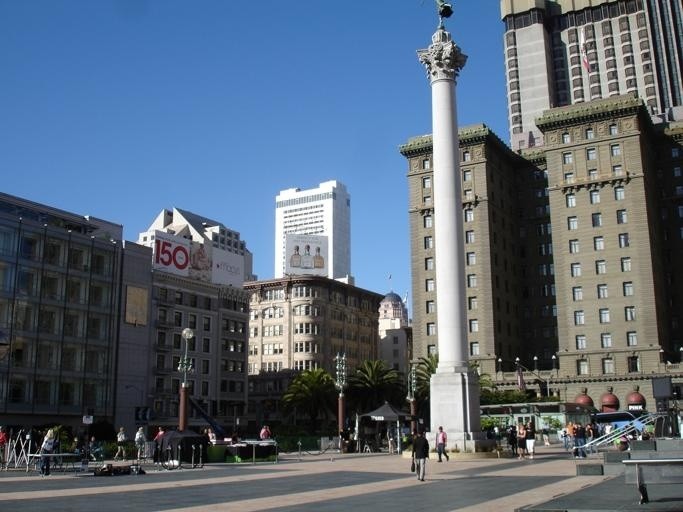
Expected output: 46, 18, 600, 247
177, 329, 194, 430
405, 363, 417, 437
334, 352, 347, 450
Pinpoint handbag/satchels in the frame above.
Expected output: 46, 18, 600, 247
411, 457, 415, 472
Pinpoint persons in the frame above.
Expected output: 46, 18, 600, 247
259, 424, 271, 440
0, 424, 8, 468
560, 421, 655, 459
411, 430, 429, 481
152, 426, 165, 465
20, 426, 61, 475
113, 427, 127, 461
193, 242, 208, 270
203, 428, 217, 446
135, 426, 146, 462
340, 426, 357, 441
388, 428, 395, 454
482, 421, 536, 461
436, 426, 449, 463
69, 431, 104, 461
542, 421, 551, 446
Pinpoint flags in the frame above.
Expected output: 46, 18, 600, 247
582, 44, 591, 75
516, 364, 527, 392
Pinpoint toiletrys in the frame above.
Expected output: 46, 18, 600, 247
290, 245, 302, 266
301, 243, 314, 269
314, 246, 324, 269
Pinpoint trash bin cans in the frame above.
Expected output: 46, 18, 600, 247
343, 440, 357, 453
